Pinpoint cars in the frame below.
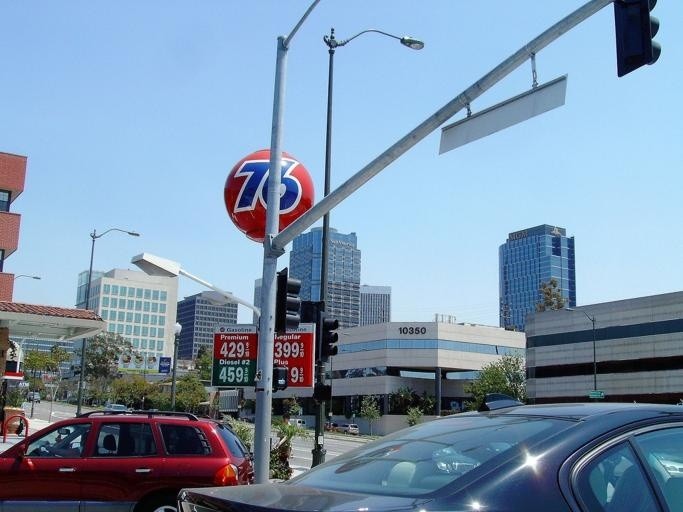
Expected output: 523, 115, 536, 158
173, 393, 683, 511
103, 403, 127, 414
26, 391, 40, 403
324, 422, 358, 435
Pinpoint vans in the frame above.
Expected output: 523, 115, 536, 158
288, 417, 305, 429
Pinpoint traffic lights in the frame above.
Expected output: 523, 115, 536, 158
313, 382, 332, 403
276, 265, 301, 337
614, 0, 661, 78
317, 312, 339, 362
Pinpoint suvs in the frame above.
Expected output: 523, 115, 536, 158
0, 410, 255, 511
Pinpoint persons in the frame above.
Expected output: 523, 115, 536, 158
609, 464, 664, 511
275, 412, 291, 466
45, 431, 88, 457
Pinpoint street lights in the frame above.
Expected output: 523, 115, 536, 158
315, 25, 426, 436
73, 225, 139, 416
169, 322, 181, 410
564, 306, 598, 403
131, 253, 279, 487
